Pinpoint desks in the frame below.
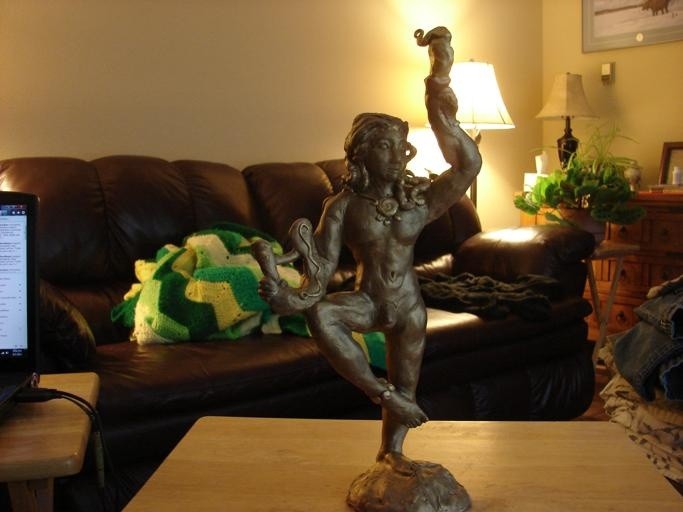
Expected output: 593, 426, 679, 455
586, 242, 640, 363
120, 416, 683, 512
0, 371, 101, 512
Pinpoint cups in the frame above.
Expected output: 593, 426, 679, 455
534, 155, 547, 174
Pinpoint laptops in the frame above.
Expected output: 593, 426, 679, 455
0, 192, 39, 424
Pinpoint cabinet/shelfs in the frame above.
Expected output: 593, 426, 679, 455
513, 191, 683, 346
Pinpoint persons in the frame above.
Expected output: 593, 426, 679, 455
250, 26, 482, 477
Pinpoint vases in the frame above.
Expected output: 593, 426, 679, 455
624, 160, 641, 191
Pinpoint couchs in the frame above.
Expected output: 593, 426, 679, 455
0, 156, 595, 469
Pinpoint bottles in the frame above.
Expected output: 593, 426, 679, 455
671, 166, 680, 185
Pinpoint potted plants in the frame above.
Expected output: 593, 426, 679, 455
513, 120, 644, 248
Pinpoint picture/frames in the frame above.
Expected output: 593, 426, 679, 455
581, 0, 683, 54
657, 142, 683, 192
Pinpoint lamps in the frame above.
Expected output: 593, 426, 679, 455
534, 72, 600, 173
449, 63, 516, 209
